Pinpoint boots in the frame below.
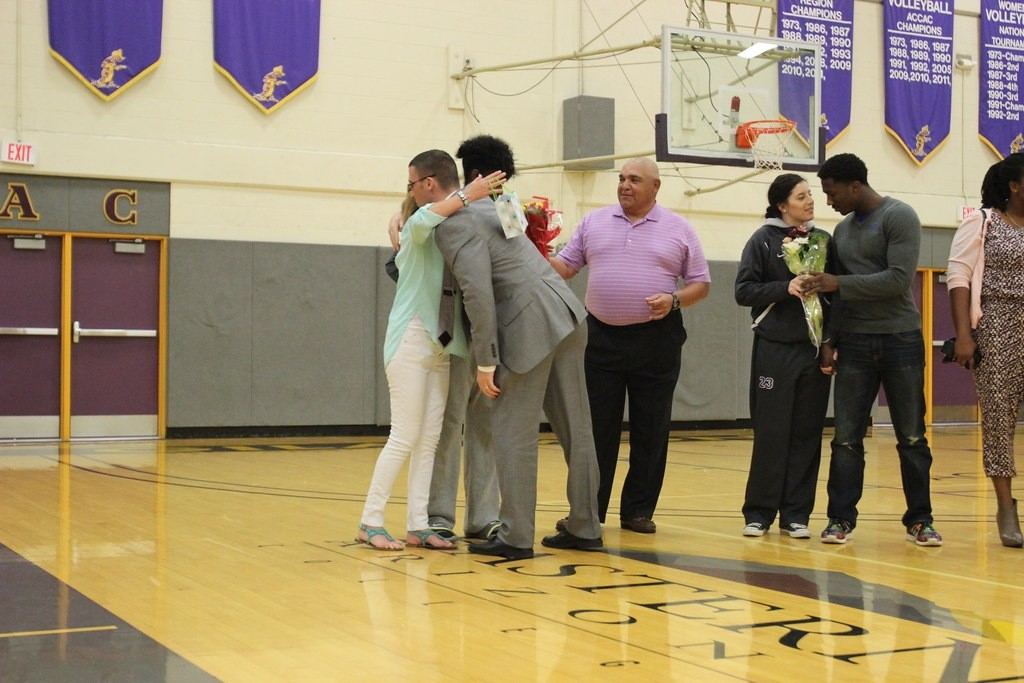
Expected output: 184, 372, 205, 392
996, 498, 1024, 548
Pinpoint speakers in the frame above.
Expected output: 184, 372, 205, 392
563, 94, 615, 171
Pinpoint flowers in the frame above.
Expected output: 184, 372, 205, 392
520, 195, 564, 261
781, 224, 831, 359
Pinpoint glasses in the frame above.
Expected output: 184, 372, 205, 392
406, 172, 438, 191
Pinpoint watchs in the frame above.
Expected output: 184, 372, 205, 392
671, 294, 680, 310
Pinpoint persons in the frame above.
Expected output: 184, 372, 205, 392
388, 150, 604, 558
355, 171, 506, 551
428, 135, 514, 541
734, 173, 837, 537
801, 154, 942, 547
947, 151, 1024, 546
549, 157, 711, 533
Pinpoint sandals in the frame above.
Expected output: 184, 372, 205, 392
354, 522, 404, 551
405, 528, 459, 550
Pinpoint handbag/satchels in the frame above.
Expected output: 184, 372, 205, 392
487, 176, 528, 240
941, 335, 983, 371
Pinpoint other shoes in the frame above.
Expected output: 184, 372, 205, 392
428, 526, 460, 544
468, 520, 501, 540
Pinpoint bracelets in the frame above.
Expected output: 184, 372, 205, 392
456, 190, 469, 208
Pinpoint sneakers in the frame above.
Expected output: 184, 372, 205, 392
779, 522, 811, 539
906, 522, 943, 547
820, 518, 854, 544
742, 522, 767, 537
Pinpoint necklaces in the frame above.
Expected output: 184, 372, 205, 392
1006, 211, 1024, 231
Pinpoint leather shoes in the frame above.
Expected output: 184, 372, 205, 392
540, 528, 604, 552
556, 517, 568, 532
621, 516, 657, 533
468, 535, 535, 559
